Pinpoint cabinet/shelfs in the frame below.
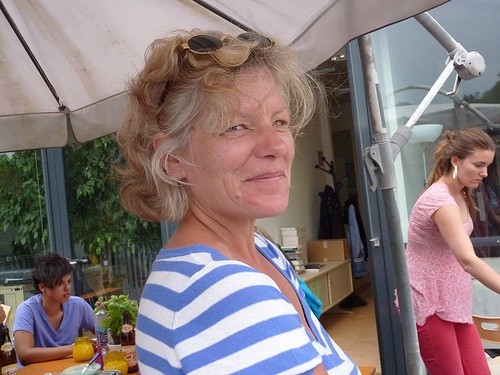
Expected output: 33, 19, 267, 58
298, 259, 354, 314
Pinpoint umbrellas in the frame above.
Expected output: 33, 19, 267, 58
0, 0, 485, 375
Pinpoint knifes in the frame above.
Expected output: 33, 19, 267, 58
81, 353, 100, 374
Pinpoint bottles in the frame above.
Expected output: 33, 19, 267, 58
121, 312, 139, 373
95, 307, 108, 353
0, 327, 17, 375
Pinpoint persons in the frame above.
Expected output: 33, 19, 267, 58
118, 29, 362, 375
395, 127, 500, 375
13, 255, 95, 368
480, 168, 500, 234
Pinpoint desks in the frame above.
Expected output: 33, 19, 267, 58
14, 345, 140, 375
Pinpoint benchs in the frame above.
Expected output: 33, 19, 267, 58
79, 287, 120, 301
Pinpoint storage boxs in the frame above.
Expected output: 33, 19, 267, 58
308, 239, 349, 262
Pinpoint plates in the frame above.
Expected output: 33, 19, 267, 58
62, 363, 101, 375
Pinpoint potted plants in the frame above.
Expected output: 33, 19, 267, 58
94, 295, 139, 346
